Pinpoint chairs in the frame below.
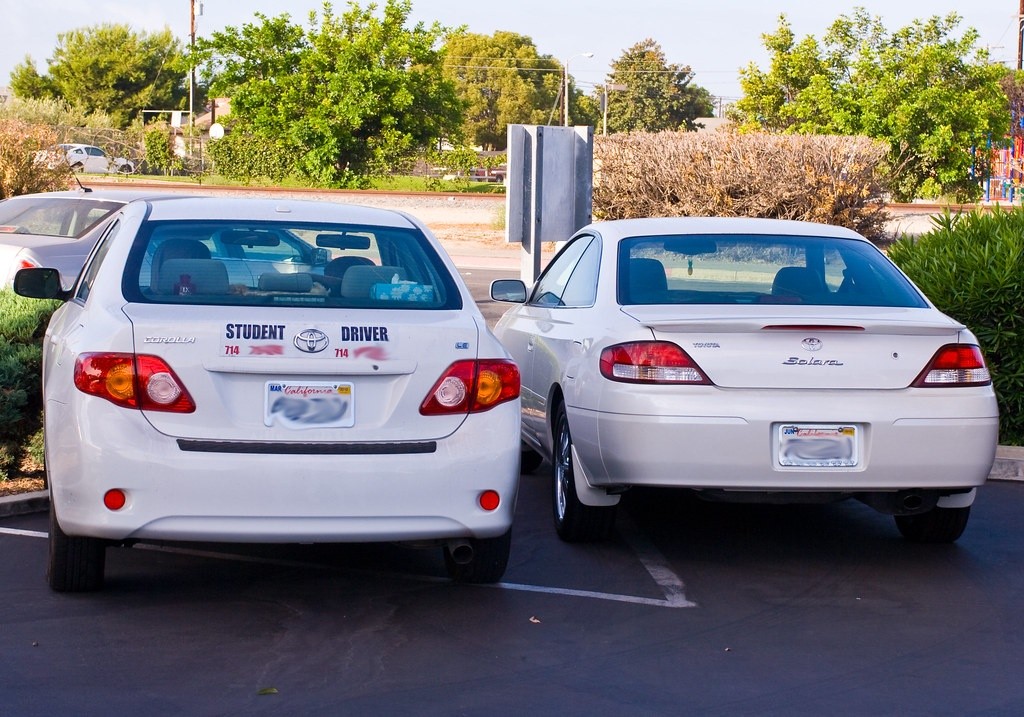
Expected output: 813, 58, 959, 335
149, 238, 212, 295
628, 256, 667, 298
772, 266, 822, 300
325, 257, 377, 293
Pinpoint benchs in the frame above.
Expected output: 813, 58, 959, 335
157, 258, 410, 300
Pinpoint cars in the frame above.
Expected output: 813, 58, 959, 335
0, 189, 331, 292
489, 216, 1000, 545
14, 194, 520, 588
37, 144, 134, 176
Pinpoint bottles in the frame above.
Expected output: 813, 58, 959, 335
174, 275, 195, 296
